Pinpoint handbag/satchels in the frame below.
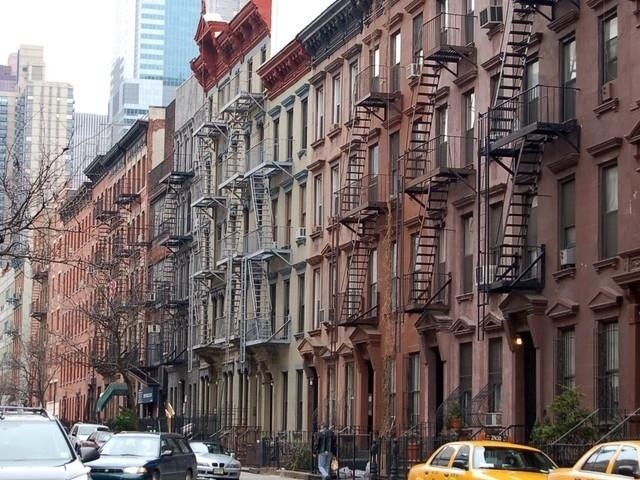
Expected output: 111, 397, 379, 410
331, 457, 339, 470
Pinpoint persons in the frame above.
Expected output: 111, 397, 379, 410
312, 421, 337, 480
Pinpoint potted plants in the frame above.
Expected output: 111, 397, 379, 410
406, 432, 424, 461
448, 402, 466, 429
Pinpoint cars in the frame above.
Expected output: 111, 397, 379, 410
0, 406, 241, 480
406, 441, 640, 480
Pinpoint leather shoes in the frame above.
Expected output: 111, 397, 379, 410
325, 476, 330, 480
